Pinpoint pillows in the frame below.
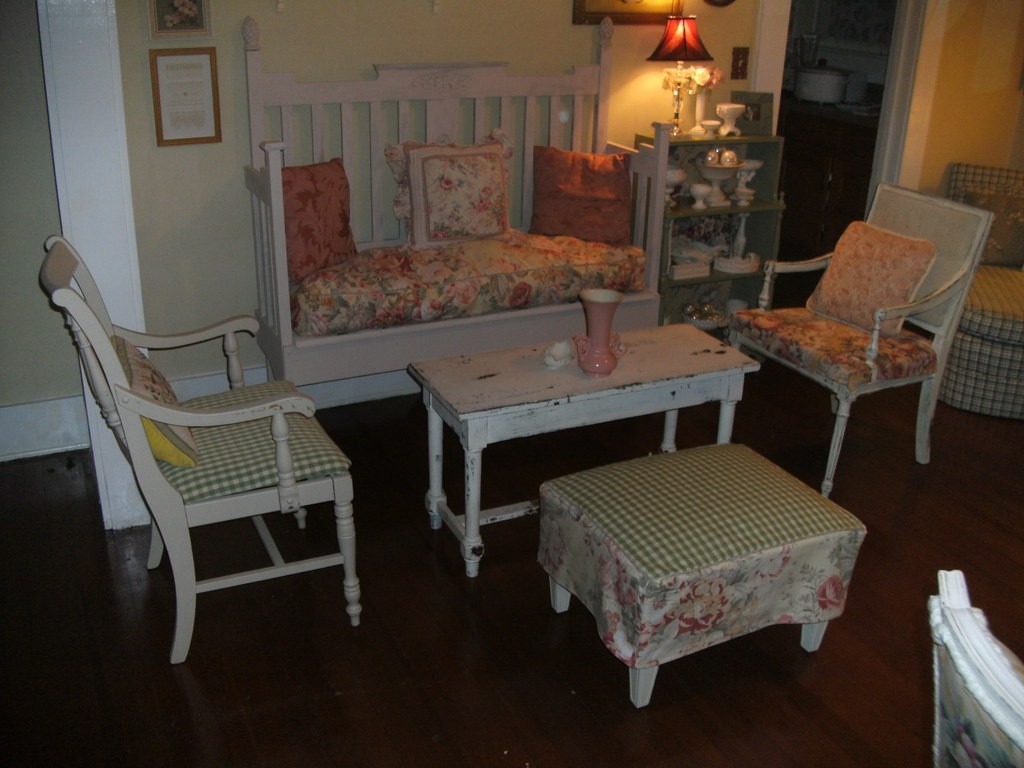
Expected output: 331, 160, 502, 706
282, 158, 359, 282
529, 145, 633, 244
965, 191, 1024, 266
806, 221, 938, 334
383, 129, 516, 249
109, 335, 197, 468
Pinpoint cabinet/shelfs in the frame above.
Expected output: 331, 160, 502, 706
776, 108, 879, 253
632, 131, 785, 330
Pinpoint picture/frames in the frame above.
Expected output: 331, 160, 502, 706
573, 0, 685, 26
146, 0, 215, 41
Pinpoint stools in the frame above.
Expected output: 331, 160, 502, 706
537, 442, 867, 709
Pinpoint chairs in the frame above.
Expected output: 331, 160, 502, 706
928, 569, 1024, 768
723, 183, 995, 499
39, 235, 361, 664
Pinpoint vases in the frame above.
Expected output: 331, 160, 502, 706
691, 91, 709, 134
572, 289, 627, 379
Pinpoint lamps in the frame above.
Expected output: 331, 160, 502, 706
646, 16, 714, 137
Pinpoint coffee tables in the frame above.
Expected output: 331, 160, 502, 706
407, 323, 761, 576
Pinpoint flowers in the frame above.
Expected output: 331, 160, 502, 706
686, 64, 726, 102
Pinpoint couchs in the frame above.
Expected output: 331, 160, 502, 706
932, 160, 1024, 422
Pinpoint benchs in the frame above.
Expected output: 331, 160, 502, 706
240, 12, 674, 412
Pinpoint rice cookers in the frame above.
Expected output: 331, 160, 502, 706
793, 58, 852, 106
